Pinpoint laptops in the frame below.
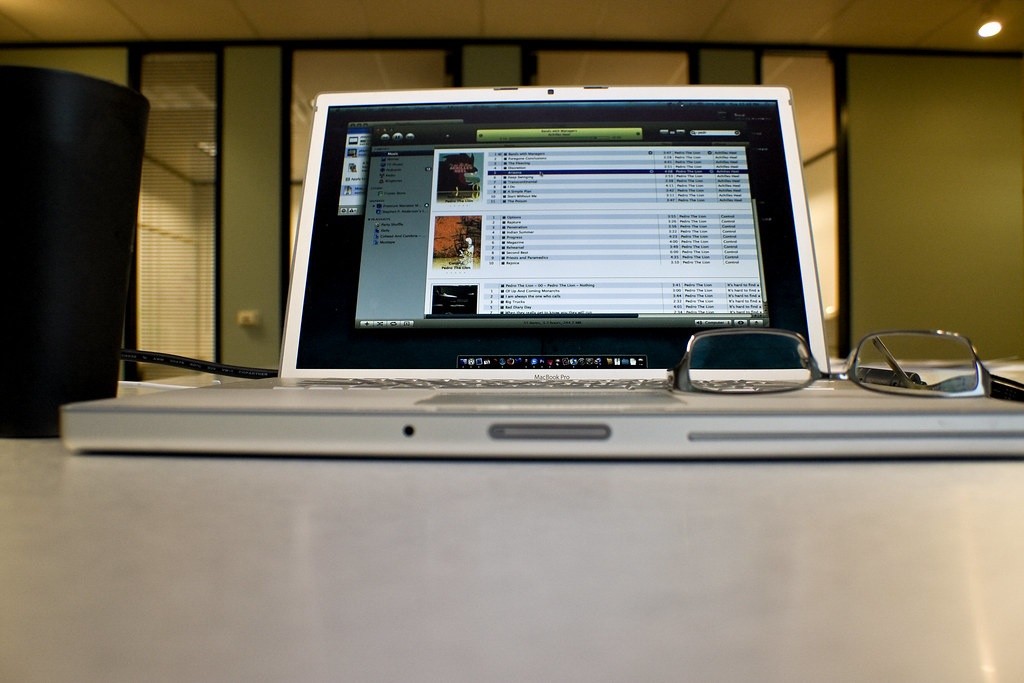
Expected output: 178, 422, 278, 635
62, 84, 1024, 465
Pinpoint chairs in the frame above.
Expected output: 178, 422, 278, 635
0, 63, 151, 438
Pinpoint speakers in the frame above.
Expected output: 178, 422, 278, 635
0, 65, 151, 435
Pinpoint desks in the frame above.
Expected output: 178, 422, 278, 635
0, 438, 1024, 683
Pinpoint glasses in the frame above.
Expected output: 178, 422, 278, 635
674, 327, 992, 399
